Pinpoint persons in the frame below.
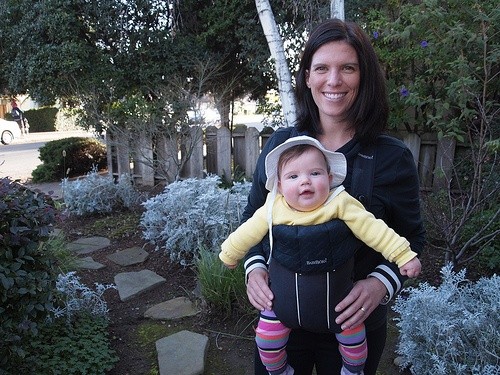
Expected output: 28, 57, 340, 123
218, 136, 422, 375
238, 16, 423, 375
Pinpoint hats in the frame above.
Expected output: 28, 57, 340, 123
264, 136, 347, 192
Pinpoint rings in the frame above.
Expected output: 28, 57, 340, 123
360, 307, 367, 314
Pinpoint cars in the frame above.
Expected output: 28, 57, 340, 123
0, 117, 21, 145
